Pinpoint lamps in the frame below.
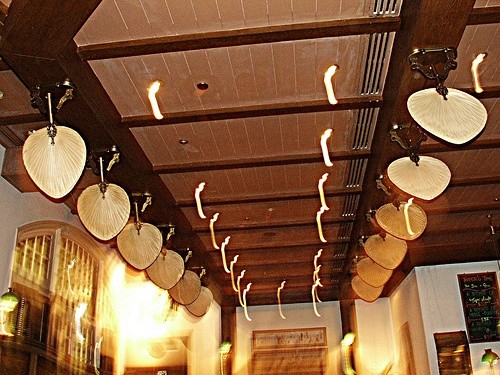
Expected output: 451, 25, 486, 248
482, 349, 499, 375
0, 287, 19, 312
